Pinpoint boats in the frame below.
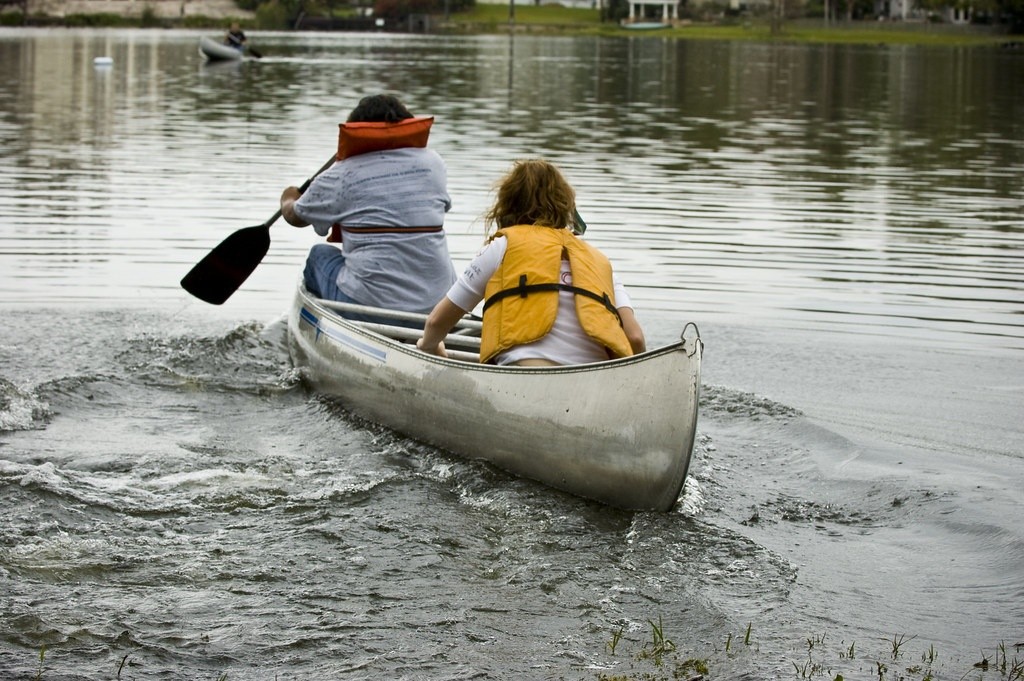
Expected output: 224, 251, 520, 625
287, 274, 705, 513
198, 34, 244, 62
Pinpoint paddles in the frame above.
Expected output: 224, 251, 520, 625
182, 151, 337, 305
225, 28, 262, 59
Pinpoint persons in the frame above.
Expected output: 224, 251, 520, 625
416, 159, 647, 366
281, 91, 460, 343
224, 22, 247, 51
